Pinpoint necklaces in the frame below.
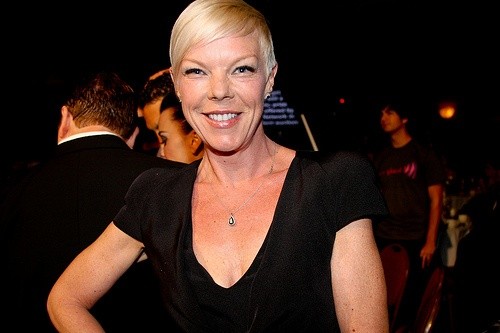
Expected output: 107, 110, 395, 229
200, 143, 276, 225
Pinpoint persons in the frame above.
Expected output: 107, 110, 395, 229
0, 68, 203, 265
47, 0, 389, 333
374, 106, 500, 333
